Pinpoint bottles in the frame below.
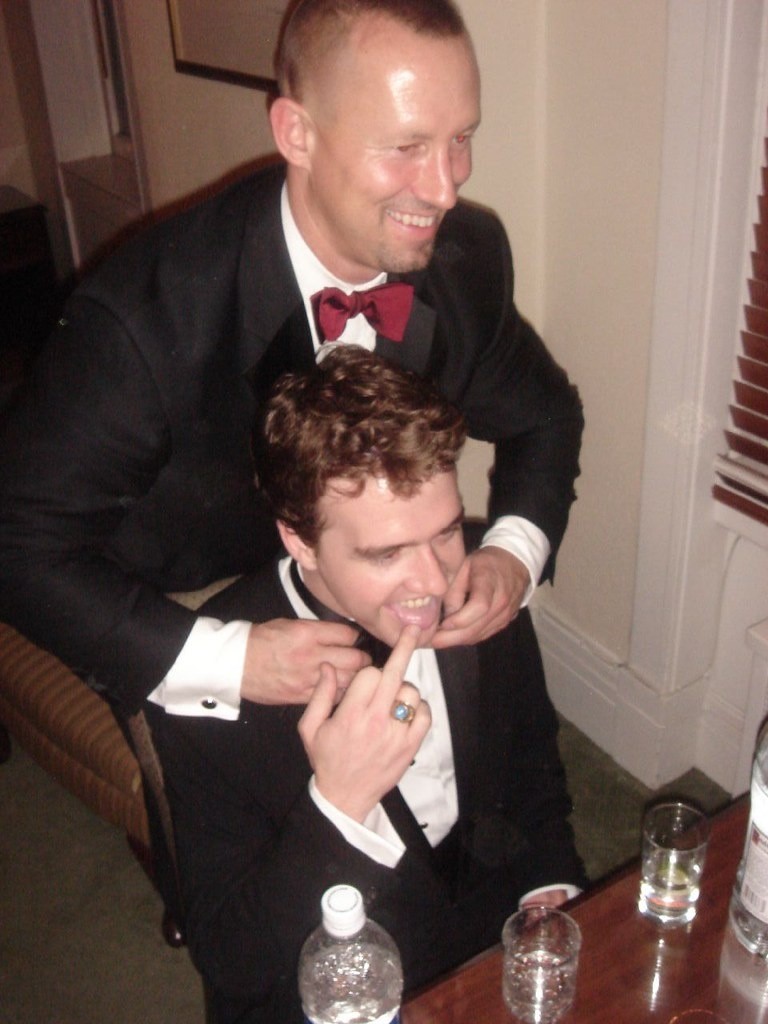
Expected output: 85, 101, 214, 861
299, 882, 404, 1024
728, 716, 768, 958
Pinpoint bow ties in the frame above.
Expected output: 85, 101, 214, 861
355, 627, 393, 668
317, 283, 415, 343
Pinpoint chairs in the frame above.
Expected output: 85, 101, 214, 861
0, 347, 195, 950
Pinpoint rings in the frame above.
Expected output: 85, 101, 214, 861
391, 700, 418, 722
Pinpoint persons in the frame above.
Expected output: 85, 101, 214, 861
143, 343, 592, 1024
3, 2, 583, 720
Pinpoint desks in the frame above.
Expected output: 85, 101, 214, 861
400, 795, 767, 1024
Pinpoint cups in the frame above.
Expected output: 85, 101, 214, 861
635, 801, 713, 940
499, 908, 580, 1024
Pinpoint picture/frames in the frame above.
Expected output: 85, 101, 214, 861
166, 1, 297, 94
